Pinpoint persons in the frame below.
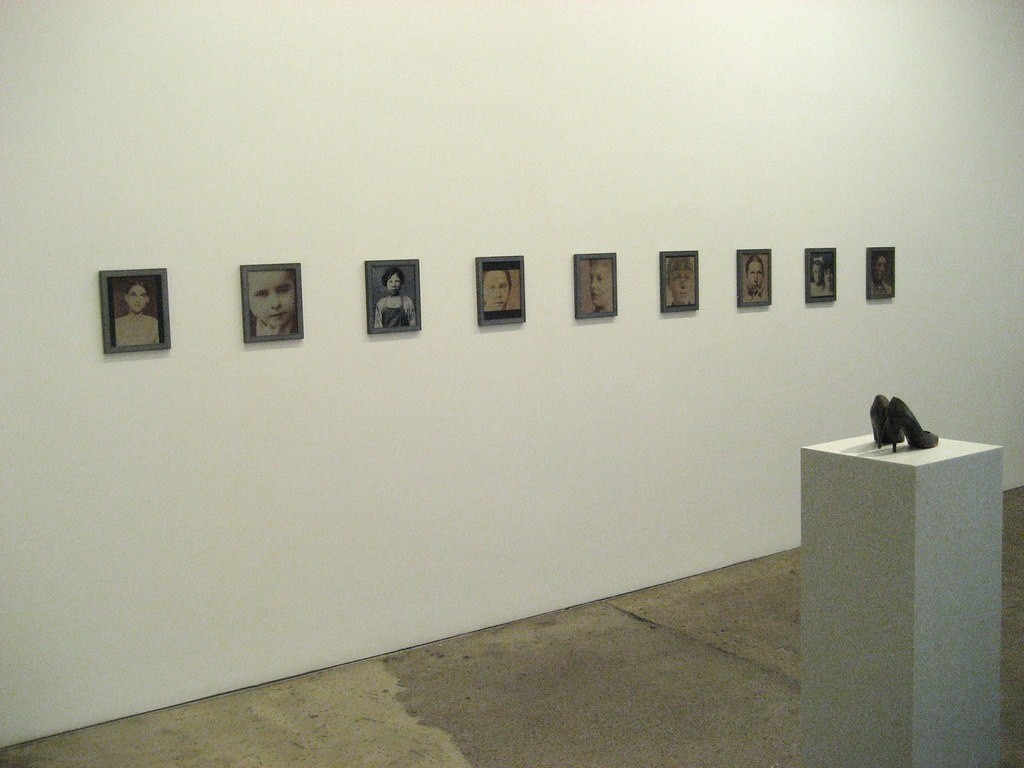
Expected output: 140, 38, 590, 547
588, 259, 614, 313
115, 279, 160, 346
821, 261, 834, 296
248, 269, 298, 335
810, 260, 824, 296
483, 270, 512, 312
373, 267, 417, 328
743, 254, 768, 302
666, 256, 694, 307
870, 251, 893, 295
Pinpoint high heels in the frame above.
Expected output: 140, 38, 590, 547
870, 395, 939, 453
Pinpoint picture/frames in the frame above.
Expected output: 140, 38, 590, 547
476, 256, 526, 326
660, 251, 699, 312
365, 259, 422, 334
737, 249, 771, 307
99, 268, 171, 353
805, 248, 836, 302
574, 253, 617, 319
866, 247, 895, 299
240, 263, 304, 343
